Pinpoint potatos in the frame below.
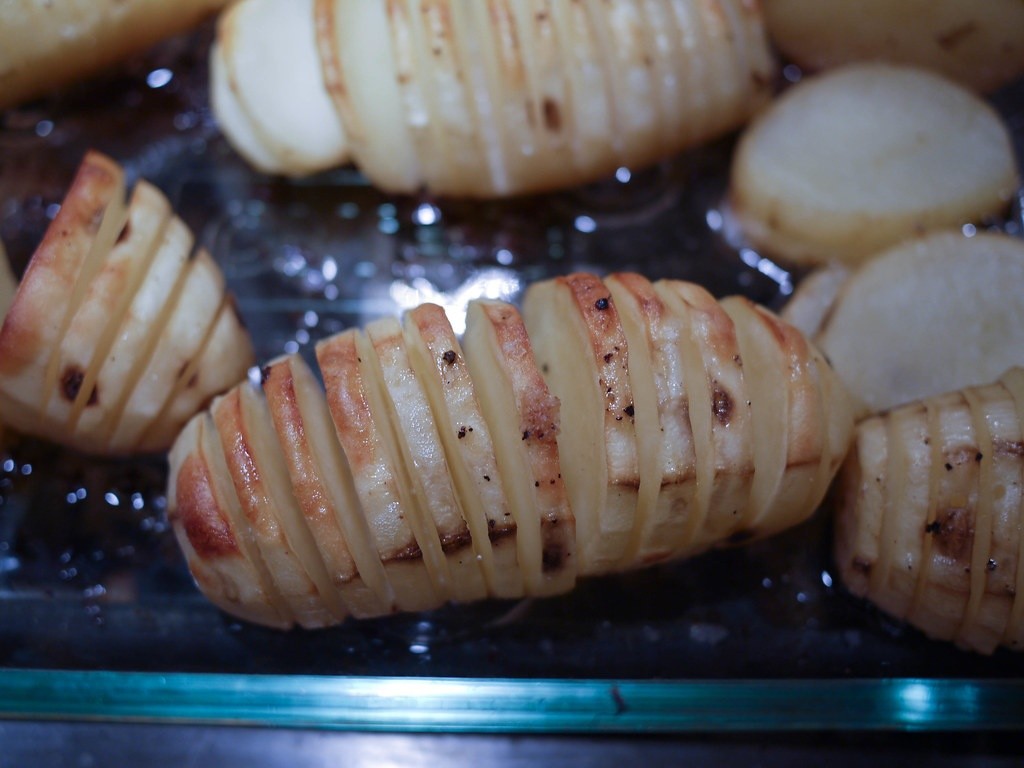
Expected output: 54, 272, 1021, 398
0, 0, 1024, 656
